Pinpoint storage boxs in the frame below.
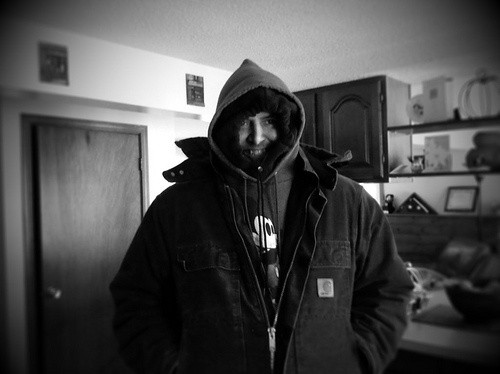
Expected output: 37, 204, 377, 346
422, 75, 454, 122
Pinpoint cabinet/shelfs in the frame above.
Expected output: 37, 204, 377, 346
292, 74, 500, 268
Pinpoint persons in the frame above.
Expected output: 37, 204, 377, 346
109, 65, 415, 374
382, 194, 394, 214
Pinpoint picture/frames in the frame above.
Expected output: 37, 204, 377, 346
443, 186, 479, 212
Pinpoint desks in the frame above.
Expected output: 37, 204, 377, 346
397, 316, 500, 368
